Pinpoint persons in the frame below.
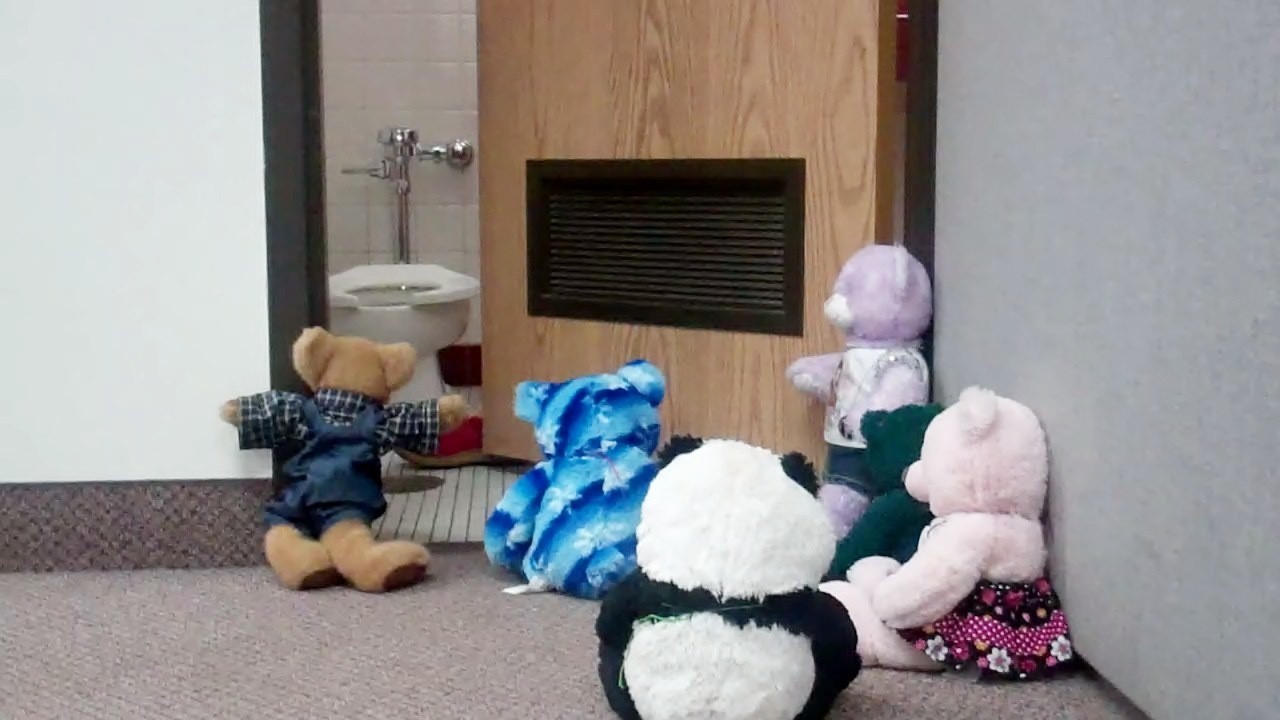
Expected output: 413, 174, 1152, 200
395, 407, 529, 466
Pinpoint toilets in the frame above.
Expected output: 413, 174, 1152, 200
323, 263, 482, 407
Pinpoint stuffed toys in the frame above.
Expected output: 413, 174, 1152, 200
595, 434, 863, 720
481, 356, 666, 602
816, 385, 1076, 684
785, 241, 935, 540
817, 400, 947, 582
218, 325, 469, 593
